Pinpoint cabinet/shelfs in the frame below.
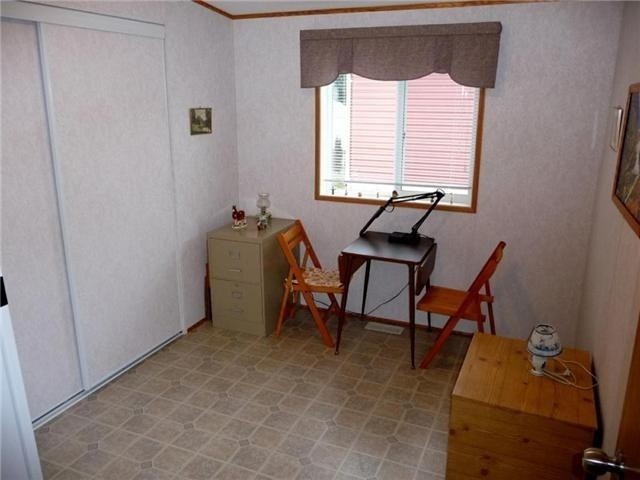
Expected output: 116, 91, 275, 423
207, 217, 299, 337
446, 332, 597, 480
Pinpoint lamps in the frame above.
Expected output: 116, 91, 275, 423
359, 189, 444, 247
256, 192, 271, 226
527, 324, 562, 377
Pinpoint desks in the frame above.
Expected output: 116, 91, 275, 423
335, 231, 438, 370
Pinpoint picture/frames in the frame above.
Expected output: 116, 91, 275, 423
611, 82, 639, 237
190, 107, 213, 136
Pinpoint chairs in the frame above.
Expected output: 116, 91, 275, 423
276, 220, 347, 348
417, 241, 506, 370
